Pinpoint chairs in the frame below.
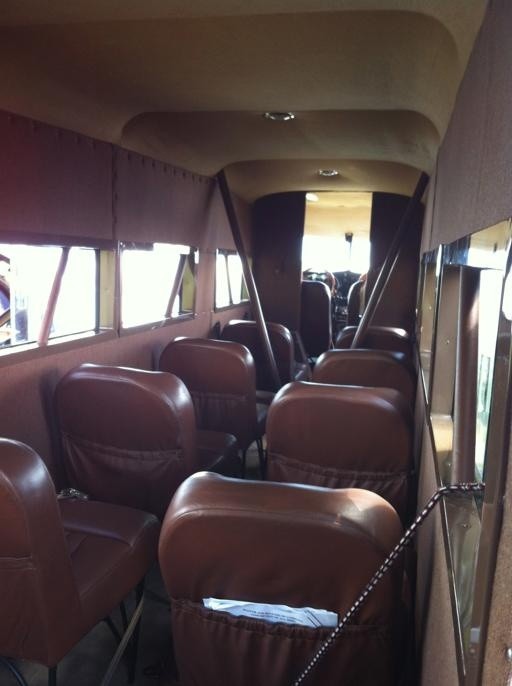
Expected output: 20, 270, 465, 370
0, 280, 417, 683
